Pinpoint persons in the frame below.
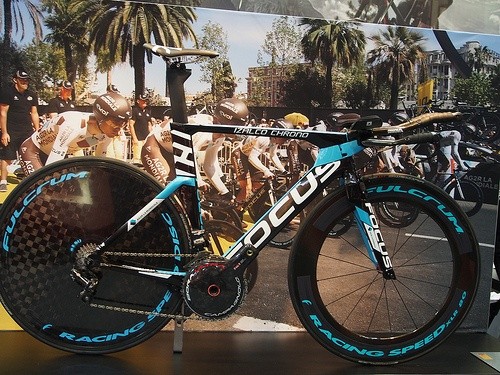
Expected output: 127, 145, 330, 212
0, 68, 40, 192
0, 79, 477, 231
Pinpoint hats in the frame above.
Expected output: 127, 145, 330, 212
138, 94, 149, 102
106, 85, 120, 93
14, 70, 31, 79
57, 80, 74, 89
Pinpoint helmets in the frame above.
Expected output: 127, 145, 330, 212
390, 111, 409, 126
214, 98, 250, 126
326, 112, 344, 128
93, 93, 132, 125
463, 123, 476, 135
271, 118, 294, 129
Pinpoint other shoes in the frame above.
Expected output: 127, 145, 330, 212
0, 180, 7, 192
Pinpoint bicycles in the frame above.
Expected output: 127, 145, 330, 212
0, 42, 482, 366
206, 113, 484, 296
